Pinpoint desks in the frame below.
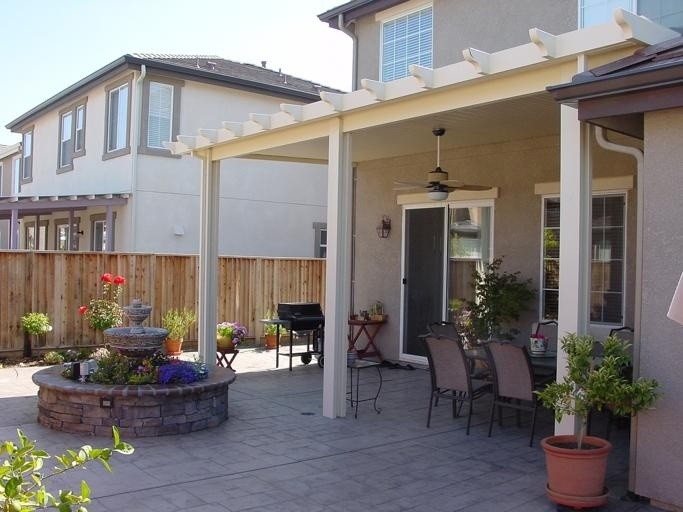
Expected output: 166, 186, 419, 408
346, 319, 387, 362
215, 349, 238, 373
344, 357, 383, 418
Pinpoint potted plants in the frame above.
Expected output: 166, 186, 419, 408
459, 253, 540, 371
531, 329, 665, 511
18, 311, 52, 347
159, 306, 195, 355
262, 324, 286, 351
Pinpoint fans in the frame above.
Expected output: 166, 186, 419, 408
391, 126, 493, 192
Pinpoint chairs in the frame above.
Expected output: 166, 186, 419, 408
419, 316, 635, 447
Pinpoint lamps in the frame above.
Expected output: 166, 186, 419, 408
374, 213, 392, 238
424, 188, 449, 201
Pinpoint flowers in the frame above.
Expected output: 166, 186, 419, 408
215, 320, 247, 347
78, 272, 125, 333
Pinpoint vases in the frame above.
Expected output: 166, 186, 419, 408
216, 336, 234, 349
